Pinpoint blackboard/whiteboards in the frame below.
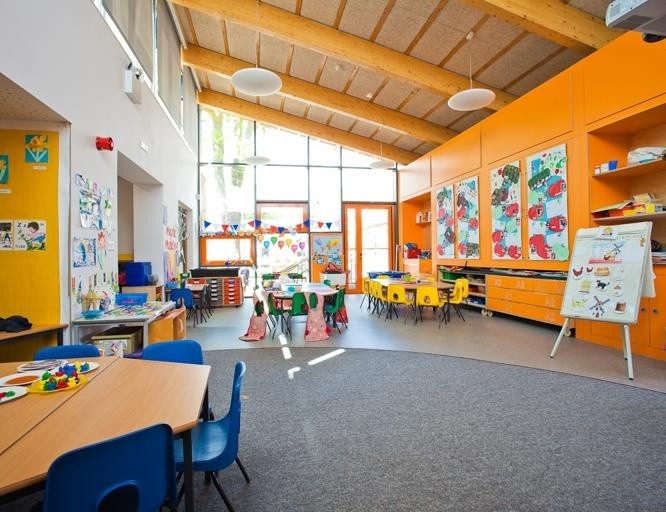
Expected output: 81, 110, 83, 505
200, 236, 255, 267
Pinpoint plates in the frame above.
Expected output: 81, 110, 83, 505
27, 375, 88, 394
0, 386, 28, 404
16, 359, 68, 372
51, 362, 99, 374
0, 372, 42, 387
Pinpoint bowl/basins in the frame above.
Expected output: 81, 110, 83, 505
281, 283, 302, 293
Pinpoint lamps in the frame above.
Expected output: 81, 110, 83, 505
449, 30, 495, 112
231, 67, 283, 98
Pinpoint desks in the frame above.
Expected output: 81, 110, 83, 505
73, 301, 186, 354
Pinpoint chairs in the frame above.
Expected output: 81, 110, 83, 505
442, 282, 466, 324
45, 424, 177, 512
368, 281, 373, 310
173, 361, 251, 512
268, 294, 291, 339
33, 346, 100, 359
187, 286, 208, 325
324, 279, 332, 286
360, 278, 381, 309
414, 286, 447, 330
254, 289, 277, 333
323, 288, 348, 334
450, 278, 470, 316
388, 284, 417, 324
286, 293, 317, 339
170, 288, 198, 329
141, 339, 215, 422
372, 281, 398, 318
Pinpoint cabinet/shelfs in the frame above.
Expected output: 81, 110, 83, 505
186, 267, 243, 311
579, 19, 665, 364
396, 150, 432, 279
433, 60, 578, 334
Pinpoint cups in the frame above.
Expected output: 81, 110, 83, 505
608, 160, 617, 171
